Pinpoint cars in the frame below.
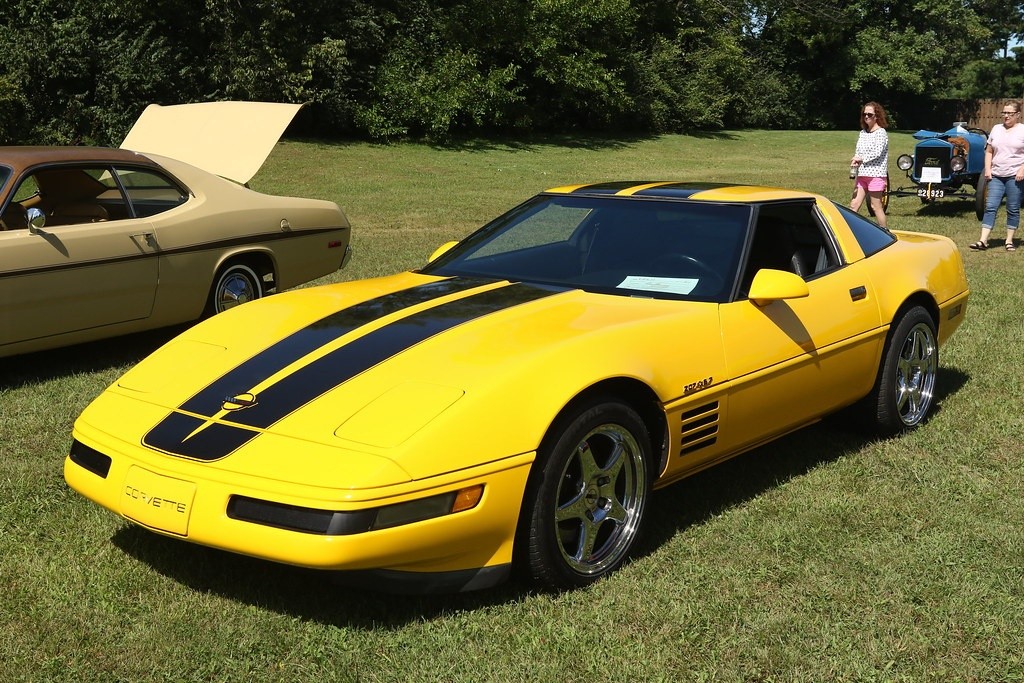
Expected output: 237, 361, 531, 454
63, 182, 973, 601
0, 100, 351, 367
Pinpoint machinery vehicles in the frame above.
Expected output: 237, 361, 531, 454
897, 120, 990, 222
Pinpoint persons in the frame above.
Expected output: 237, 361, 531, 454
969, 99, 1024, 251
846, 102, 890, 233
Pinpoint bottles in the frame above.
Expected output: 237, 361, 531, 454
849, 163, 856, 179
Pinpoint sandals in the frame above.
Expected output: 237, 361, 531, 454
1006, 243, 1016, 252
970, 241, 988, 250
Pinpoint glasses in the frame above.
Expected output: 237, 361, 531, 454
1000, 112, 1017, 116
862, 113, 876, 118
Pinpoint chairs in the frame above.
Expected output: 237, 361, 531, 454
52, 203, 109, 226
0, 202, 27, 232
